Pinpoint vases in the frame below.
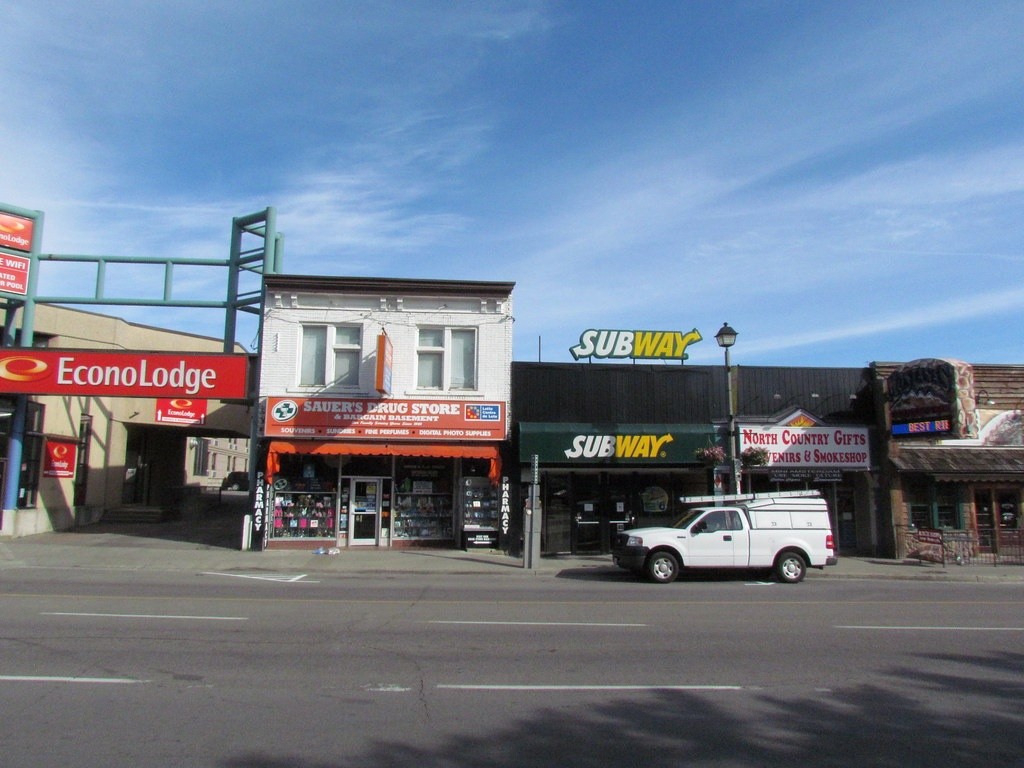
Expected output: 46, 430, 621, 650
705, 460, 713, 468
743, 460, 753, 465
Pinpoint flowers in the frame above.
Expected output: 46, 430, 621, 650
738, 447, 771, 469
692, 446, 727, 470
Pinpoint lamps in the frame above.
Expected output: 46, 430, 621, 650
975, 390, 996, 406
766, 393, 820, 425
737, 393, 780, 417
812, 392, 857, 416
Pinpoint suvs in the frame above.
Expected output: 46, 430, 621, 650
611, 488, 840, 584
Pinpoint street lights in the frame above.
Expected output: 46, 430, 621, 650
716, 322, 741, 495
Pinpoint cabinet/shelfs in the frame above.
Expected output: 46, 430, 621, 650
542, 506, 571, 551
393, 493, 453, 539
463, 477, 500, 531
273, 491, 337, 538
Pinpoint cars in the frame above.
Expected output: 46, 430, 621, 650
224, 471, 249, 492
